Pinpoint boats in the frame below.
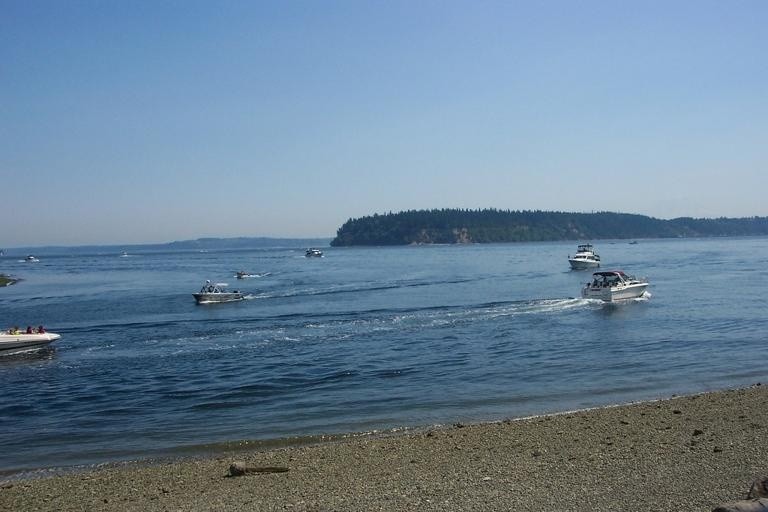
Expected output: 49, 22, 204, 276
237, 271, 272, 278
21, 255, 40, 263
582, 270, 649, 302
0, 329, 61, 356
305, 247, 324, 257
192, 279, 244, 305
568, 242, 600, 269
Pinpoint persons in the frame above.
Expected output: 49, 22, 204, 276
36, 324, 44, 333
9, 327, 16, 335
13, 326, 20, 335
26, 325, 33, 333
